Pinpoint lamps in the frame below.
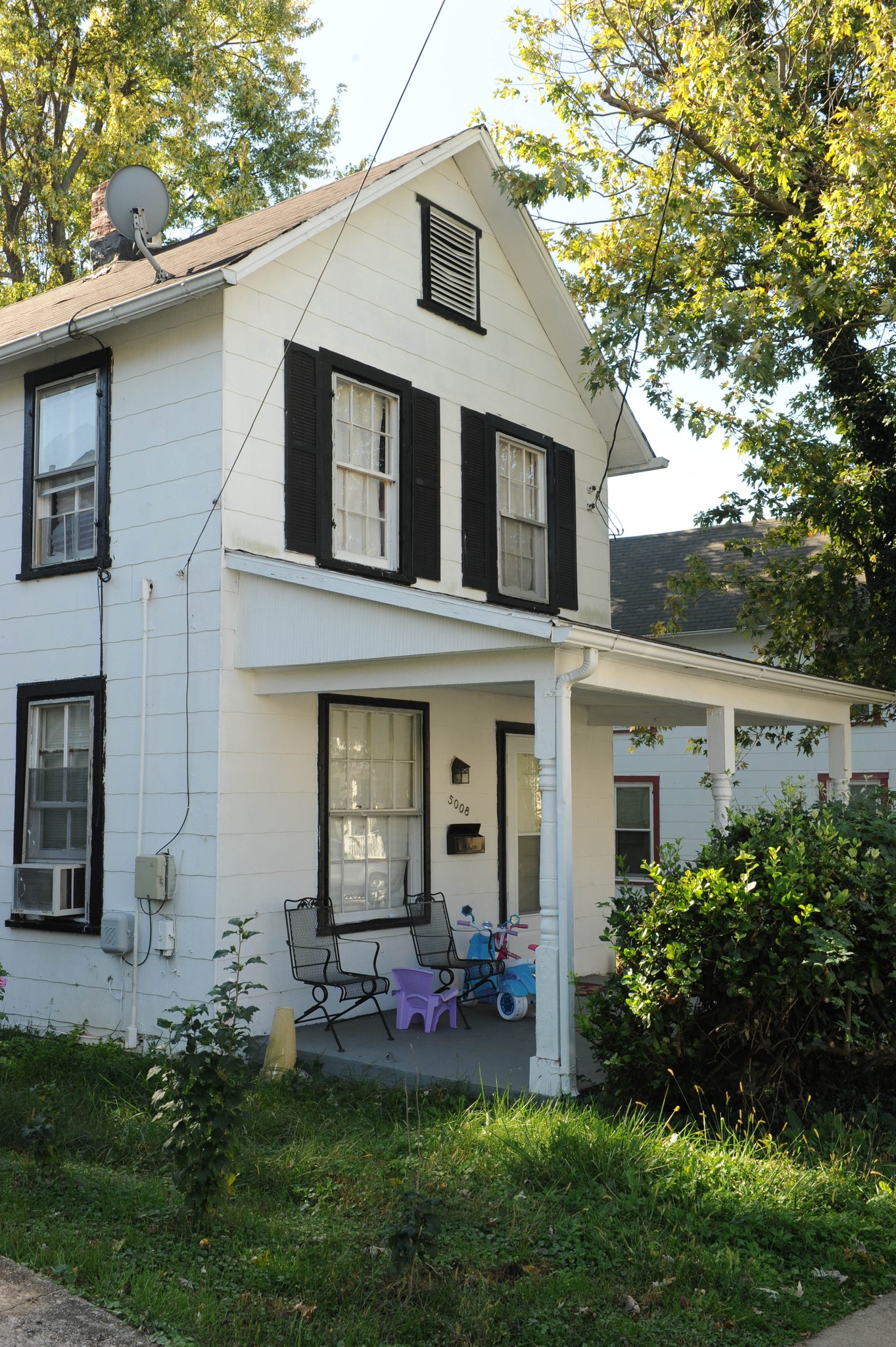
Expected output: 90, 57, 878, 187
452, 757, 471, 784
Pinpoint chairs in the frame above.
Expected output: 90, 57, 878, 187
391, 968, 458, 1033
284, 897, 395, 1052
405, 892, 505, 1030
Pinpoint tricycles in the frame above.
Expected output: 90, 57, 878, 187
457, 904, 539, 1022
